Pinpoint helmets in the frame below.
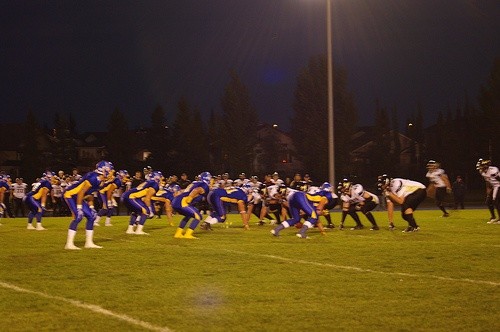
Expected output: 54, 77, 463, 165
350, 184, 364, 199
378, 174, 390, 191
426, 160, 441, 171
0, 169, 351, 196
476, 157, 492, 170
95, 160, 112, 178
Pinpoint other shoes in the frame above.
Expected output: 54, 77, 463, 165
444, 212, 450, 216
403, 225, 420, 233
356, 224, 378, 230
135, 210, 335, 230
340, 224, 358, 230
486, 218, 500, 225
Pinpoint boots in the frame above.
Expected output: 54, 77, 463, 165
296, 225, 309, 239
93, 215, 113, 226
63, 229, 82, 249
174, 227, 195, 239
27, 222, 46, 230
84, 230, 103, 248
125, 224, 148, 234
270, 224, 284, 237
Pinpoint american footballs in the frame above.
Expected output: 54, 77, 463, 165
294, 221, 303, 229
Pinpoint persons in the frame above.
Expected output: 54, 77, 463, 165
340, 177, 380, 230
0, 169, 321, 223
172, 171, 211, 239
453, 176, 464, 209
307, 182, 338, 229
200, 184, 249, 231
22, 175, 55, 230
271, 190, 329, 240
426, 160, 452, 217
63, 161, 115, 249
477, 160, 500, 224
91, 170, 123, 227
136, 184, 179, 227
120, 170, 158, 235
378, 174, 426, 232
